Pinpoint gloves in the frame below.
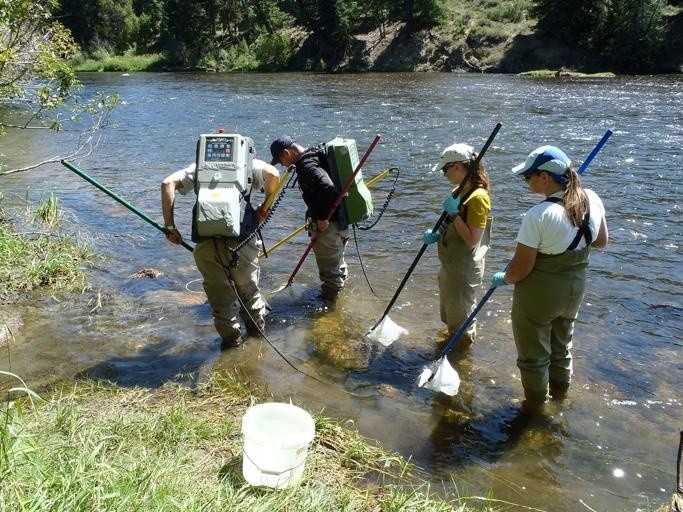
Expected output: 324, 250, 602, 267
443, 193, 460, 215
422, 229, 439, 243
492, 272, 506, 285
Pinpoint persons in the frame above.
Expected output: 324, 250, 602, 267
420, 140, 492, 348
487, 146, 609, 403
268, 136, 349, 302
159, 135, 280, 350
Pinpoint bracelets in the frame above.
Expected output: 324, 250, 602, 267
164, 224, 175, 230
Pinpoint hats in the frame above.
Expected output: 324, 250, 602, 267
270, 135, 295, 166
511, 146, 571, 179
432, 144, 474, 172
244, 136, 255, 153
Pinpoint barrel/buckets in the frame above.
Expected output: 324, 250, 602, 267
240, 401, 315, 490
